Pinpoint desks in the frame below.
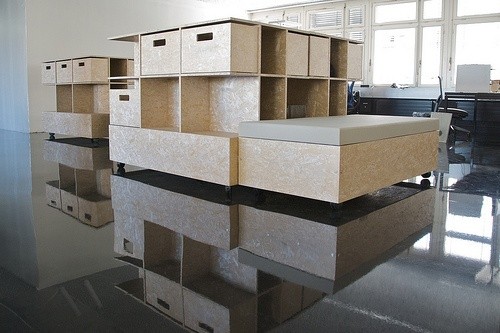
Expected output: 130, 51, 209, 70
445, 92, 500, 127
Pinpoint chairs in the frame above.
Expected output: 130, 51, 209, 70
435, 76, 470, 140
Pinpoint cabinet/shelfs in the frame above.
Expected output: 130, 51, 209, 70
40, 57, 133, 147
108, 17, 363, 194
111, 163, 328, 333
43, 138, 114, 228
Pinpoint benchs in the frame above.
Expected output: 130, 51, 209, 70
238, 114, 438, 210
239, 181, 435, 296
